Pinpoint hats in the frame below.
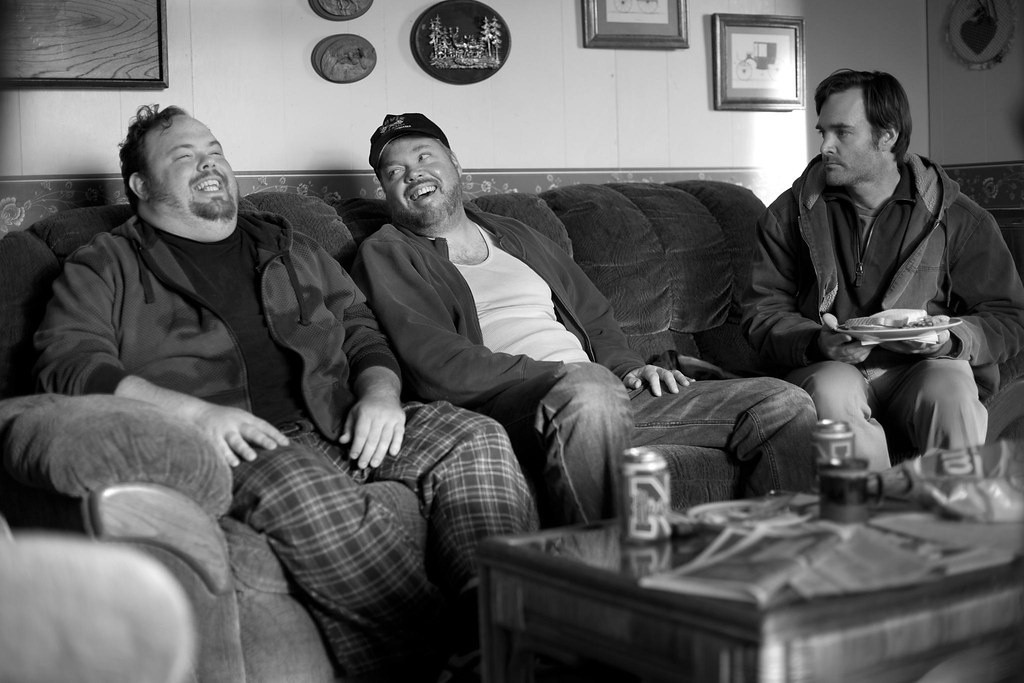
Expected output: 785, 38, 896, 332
368, 112, 451, 171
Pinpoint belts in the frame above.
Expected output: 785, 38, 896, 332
277, 418, 314, 439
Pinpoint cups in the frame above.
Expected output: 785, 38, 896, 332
817, 458, 885, 518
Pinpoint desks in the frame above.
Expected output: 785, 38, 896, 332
472, 485, 1023, 683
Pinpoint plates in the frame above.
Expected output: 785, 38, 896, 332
686, 501, 818, 532
835, 316, 962, 341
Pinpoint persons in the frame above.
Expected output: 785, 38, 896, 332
362, 114, 819, 527
741, 69, 1024, 467
35, 103, 538, 683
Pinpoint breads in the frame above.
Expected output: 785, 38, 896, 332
846, 308, 927, 331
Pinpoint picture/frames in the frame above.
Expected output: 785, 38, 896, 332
581, 0, 691, 49
711, 13, 808, 112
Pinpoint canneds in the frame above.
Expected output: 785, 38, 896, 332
811, 420, 853, 493
617, 447, 671, 543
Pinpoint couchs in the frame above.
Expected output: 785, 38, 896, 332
1, 181, 768, 683
